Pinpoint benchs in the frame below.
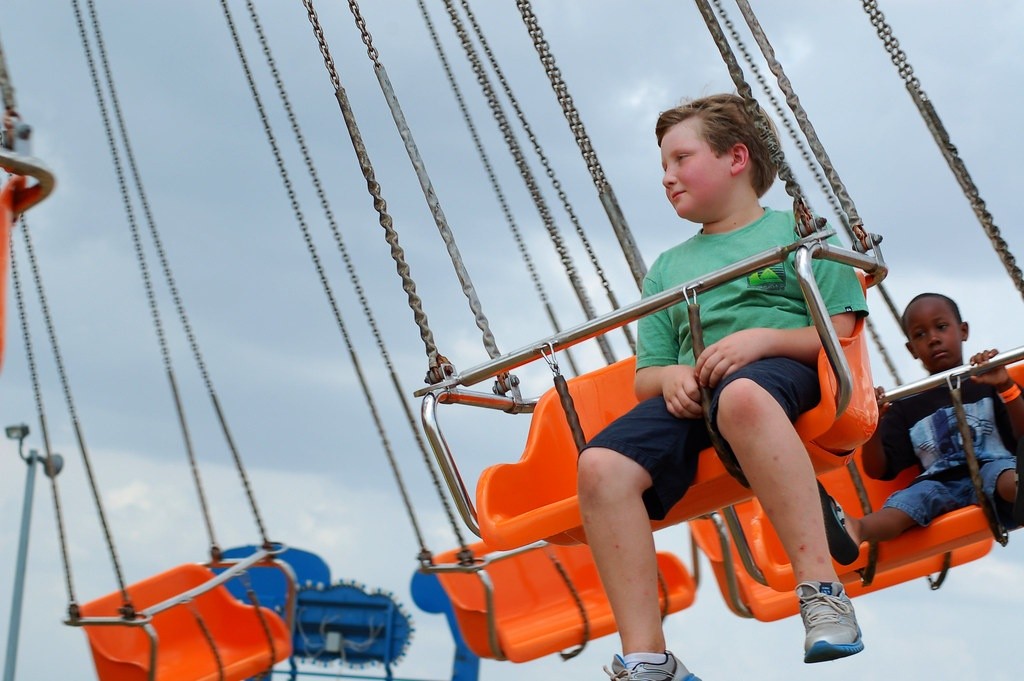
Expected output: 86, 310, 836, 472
62, 558, 299, 681
729, 340, 1024, 592
688, 500, 993, 623
431, 539, 697, 663
411, 565, 482, 680
412, 218, 890, 554
200, 544, 416, 680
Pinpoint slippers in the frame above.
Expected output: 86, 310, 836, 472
814, 478, 859, 566
1013, 435, 1024, 526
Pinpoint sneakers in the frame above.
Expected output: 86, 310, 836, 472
606, 650, 700, 680
798, 581, 863, 664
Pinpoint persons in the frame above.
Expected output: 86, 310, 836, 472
816, 293, 1024, 566
576, 92, 870, 680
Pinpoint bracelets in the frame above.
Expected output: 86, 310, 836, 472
997, 382, 1021, 404
